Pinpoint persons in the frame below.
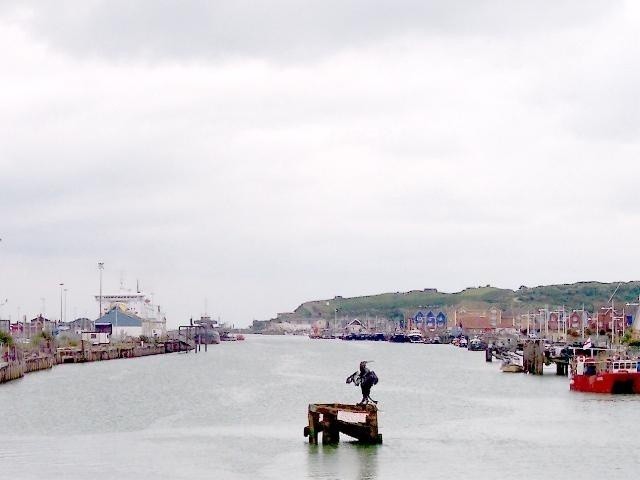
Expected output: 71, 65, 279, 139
545, 335, 640, 377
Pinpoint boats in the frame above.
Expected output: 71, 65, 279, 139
569, 351, 640, 395
